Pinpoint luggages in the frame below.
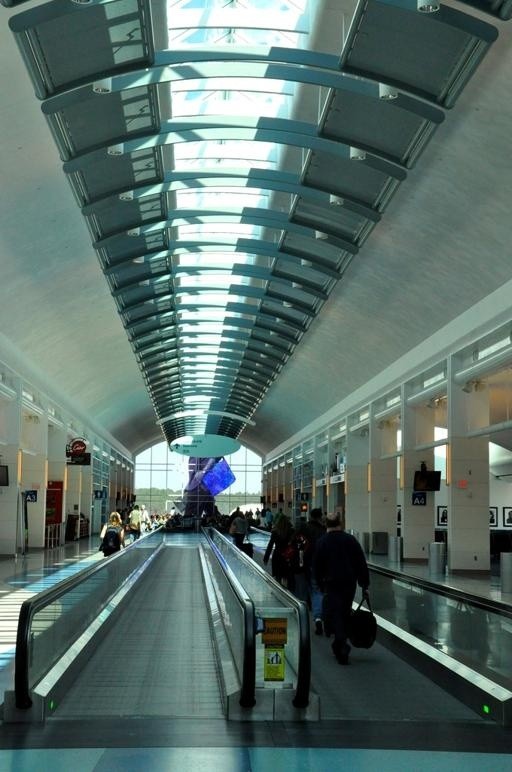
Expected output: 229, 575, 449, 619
243, 539, 253, 558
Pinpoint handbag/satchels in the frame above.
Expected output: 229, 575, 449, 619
125, 524, 133, 532
348, 596, 377, 649
229, 522, 236, 535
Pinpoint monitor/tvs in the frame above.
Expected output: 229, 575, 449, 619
0, 465, 9, 486
413, 470, 441, 492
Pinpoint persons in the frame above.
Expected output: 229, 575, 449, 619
261, 507, 334, 639
98, 510, 127, 593
311, 511, 370, 666
489, 510, 494, 523
440, 509, 447, 524
506, 510, 511, 525
128, 503, 285, 551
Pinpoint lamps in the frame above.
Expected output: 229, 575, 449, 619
379, 82, 399, 102
417, 0, 440, 15
119, 190, 134, 202
73, 0, 94, 6
92, 77, 113, 94
222, 229, 329, 435
329, 195, 344, 208
134, 255, 179, 439
107, 143, 125, 156
349, 146, 367, 161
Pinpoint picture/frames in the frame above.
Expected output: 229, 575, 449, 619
437, 504, 449, 526
489, 505, 498, 527
503, 506, 512, 527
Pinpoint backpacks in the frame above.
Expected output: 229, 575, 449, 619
99, 524, 123, 556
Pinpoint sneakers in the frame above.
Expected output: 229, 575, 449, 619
314, 618, 323, 636
335, 654, 349, 665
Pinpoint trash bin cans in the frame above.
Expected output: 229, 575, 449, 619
387, 536, 401, 562
500, 552, 511, 593
428, 542, 447, 579
